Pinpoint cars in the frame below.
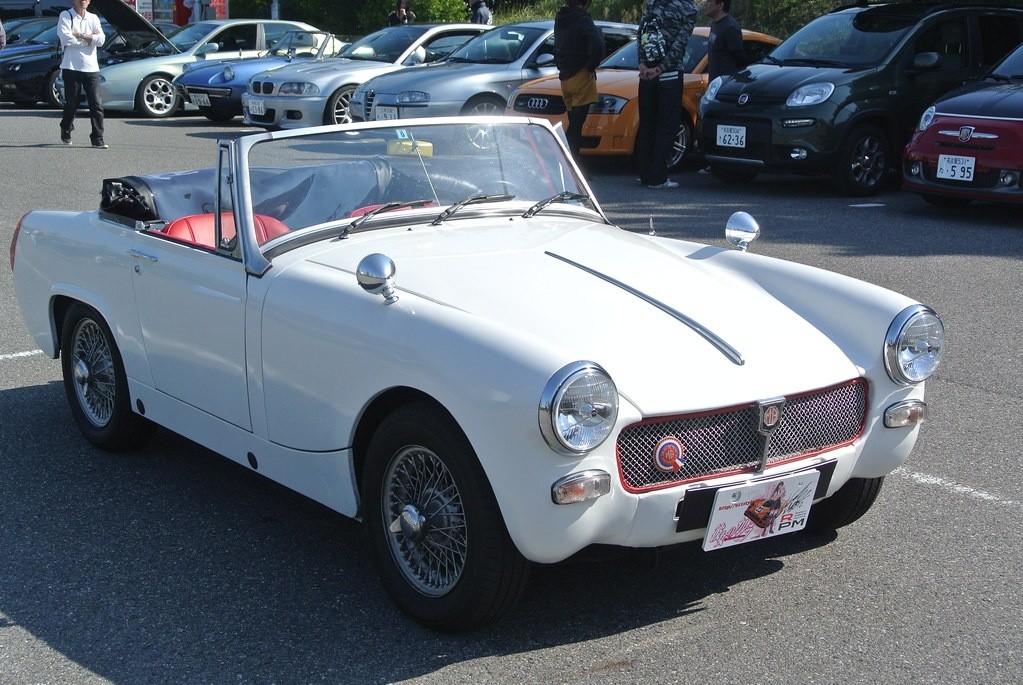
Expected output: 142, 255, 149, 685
0, 0, 1023, 214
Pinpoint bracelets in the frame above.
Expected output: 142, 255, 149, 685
80, 33, 84, 38
655, 68, 660, 74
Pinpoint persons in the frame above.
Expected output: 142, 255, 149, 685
468, 0, 492, 25
57, 0, 108, 149
636, 0, 698, 188
388, 0, 416, 27
703, 0, 753, 86
553, 0, 605, 181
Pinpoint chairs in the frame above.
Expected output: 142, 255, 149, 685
159, 212, 292, 249
933, 23, 968, 67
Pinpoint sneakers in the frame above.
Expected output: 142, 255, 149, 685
92, 136, 108, 149
60, 127, 72, 145
648, 179, 679, 189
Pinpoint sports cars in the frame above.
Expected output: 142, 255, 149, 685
9, 115, 946, 635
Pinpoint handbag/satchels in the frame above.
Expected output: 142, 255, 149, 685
51, 53, 64, 69
